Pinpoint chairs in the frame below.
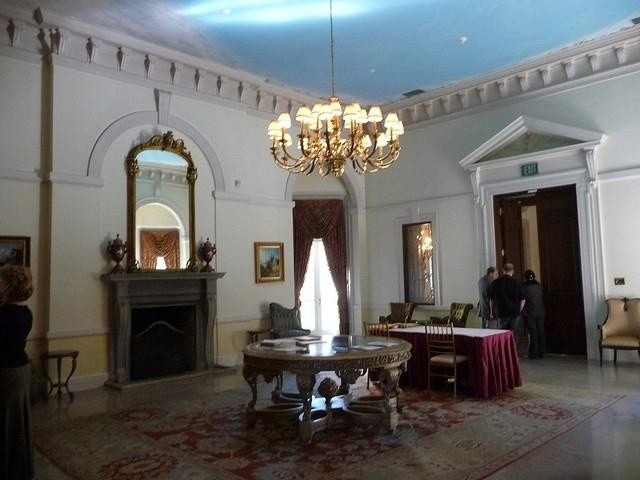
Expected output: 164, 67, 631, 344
363, 321, 389, 389
595, 296, 640, 366
378, 302, 417, 323
422, 321, 471, 397
428, 302, 475, 326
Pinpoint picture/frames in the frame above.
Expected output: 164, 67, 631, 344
254, 241, 284, 283
1, 234, 31, 271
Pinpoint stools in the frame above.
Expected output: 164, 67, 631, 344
38, 348, 79, 404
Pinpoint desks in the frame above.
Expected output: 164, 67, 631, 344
374, 322, 523, 399
247, 328, 270, 344
242, 334, 413, 446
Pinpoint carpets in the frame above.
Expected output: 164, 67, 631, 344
34, 379, 627, 480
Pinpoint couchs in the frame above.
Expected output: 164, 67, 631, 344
267, 302, 311, 337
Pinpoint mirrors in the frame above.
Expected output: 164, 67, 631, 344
126, 131, 196, 270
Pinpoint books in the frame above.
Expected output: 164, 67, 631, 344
260, 332, 399, 352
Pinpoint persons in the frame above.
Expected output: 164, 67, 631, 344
0, 262, 36, 479
518, 270, 548, 361
475, 266, 498, 328
489, 262, 527, 341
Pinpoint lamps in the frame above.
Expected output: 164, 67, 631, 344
264, 0, 405, 181
